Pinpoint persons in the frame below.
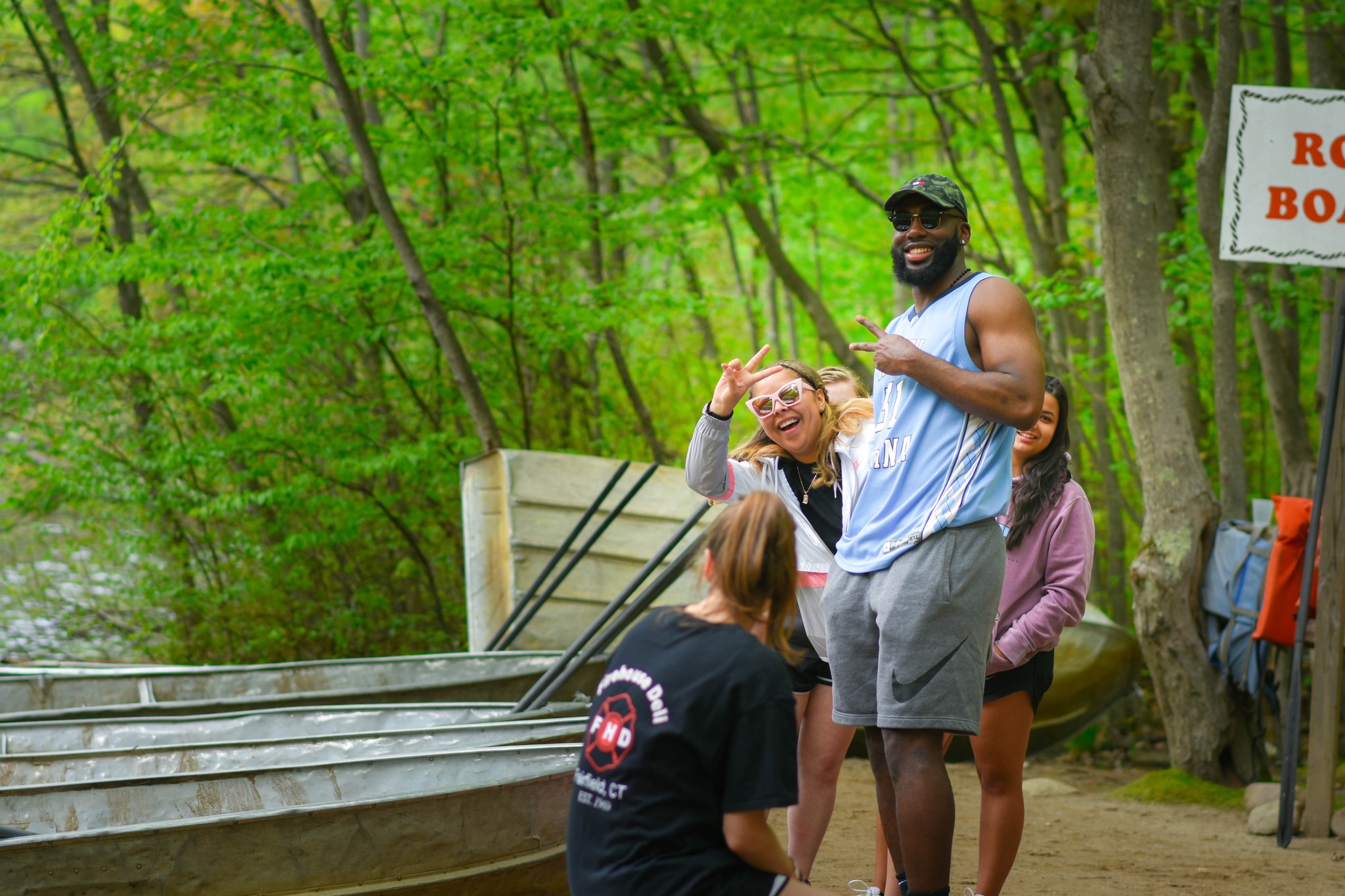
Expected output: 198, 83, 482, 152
681, 344, 890, 896
565, 491, 816, 896
819, 176, 1044, 896
854, 378, 1093, 896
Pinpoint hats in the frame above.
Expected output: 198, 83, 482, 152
884, 173, 968, 223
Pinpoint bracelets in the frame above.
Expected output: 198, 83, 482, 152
789, 854, 802, 880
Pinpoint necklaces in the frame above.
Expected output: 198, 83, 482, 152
796, 464, 819, 504
947, 268, 971, 289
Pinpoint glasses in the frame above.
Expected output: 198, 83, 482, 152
888, 210, 963, 233
744, 377, 816, 419
